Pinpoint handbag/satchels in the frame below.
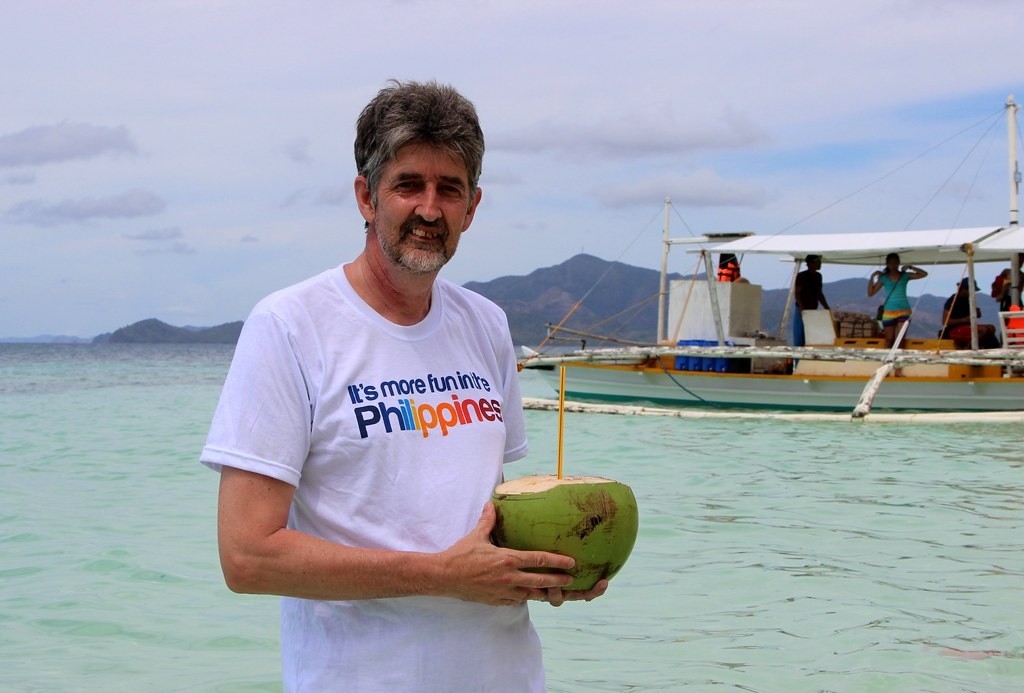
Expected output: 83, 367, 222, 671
876, 304, 884, 320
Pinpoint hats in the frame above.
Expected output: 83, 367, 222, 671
957, 277, 980, 291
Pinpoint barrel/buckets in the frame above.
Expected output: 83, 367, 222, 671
675, 340, 729, 372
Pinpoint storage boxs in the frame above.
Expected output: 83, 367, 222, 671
674, 340, 733, 372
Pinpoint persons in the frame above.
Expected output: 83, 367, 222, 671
943, 277, 1000, 350
868, 253, 928, 349
199, 79, 608, 693
793, 254, 831, 347
992, 252, 1024, 327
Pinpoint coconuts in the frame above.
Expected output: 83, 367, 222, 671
490, 473, 638, 592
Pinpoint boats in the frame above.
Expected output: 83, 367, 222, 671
517, 95, 1024, 424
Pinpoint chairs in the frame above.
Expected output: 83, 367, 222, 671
999, 311, 1024, 377
